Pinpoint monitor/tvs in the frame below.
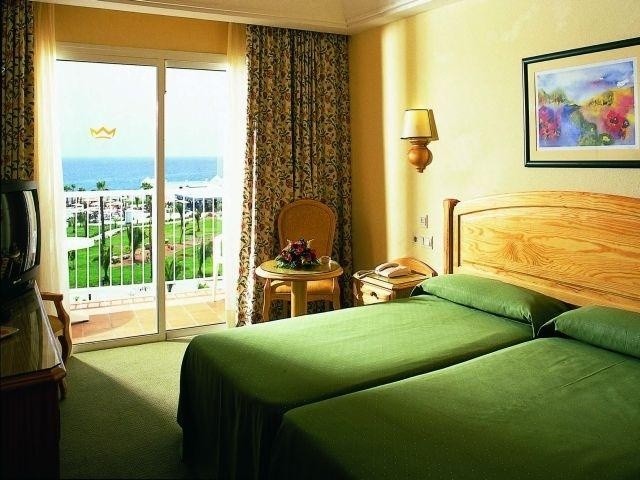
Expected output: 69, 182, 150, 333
0, 181, 43, 283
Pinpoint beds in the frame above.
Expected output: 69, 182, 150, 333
175, 192, 640, 480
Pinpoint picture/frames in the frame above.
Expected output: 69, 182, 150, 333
521, 37, 640, 170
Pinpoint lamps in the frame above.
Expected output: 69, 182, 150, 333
401, 109, 434, 174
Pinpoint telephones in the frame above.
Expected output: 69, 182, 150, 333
375, 262, 409, 278
